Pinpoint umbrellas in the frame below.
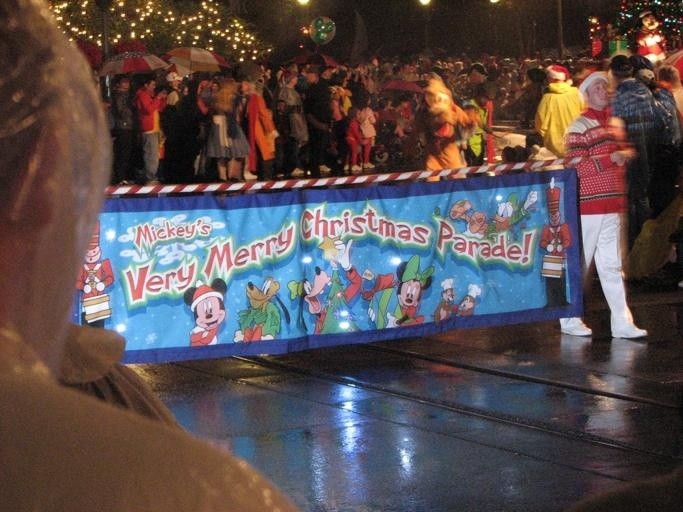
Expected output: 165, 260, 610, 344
73, 33, 235, 77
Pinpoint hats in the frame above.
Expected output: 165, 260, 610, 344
578, 72, 609, 93
544, 63, 573, 85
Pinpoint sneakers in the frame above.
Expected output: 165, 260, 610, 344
560, 323, 593, 336
612, 325, 648, 338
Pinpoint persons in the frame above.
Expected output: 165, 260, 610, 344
110, 62, 305, 185
304, 50, 683, 242
558, 68, 648, 342
1, 0, 305, 510
